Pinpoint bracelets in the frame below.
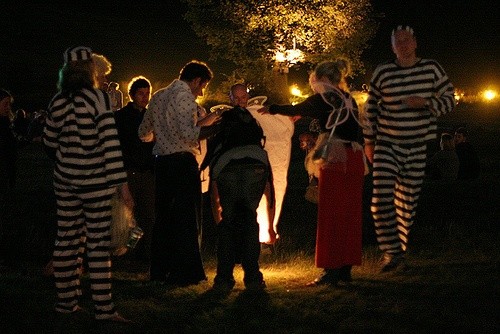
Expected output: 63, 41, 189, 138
425, 98, 430, 108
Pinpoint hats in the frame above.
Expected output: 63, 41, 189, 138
64, 46, 92, 63
391, 25, 415, 36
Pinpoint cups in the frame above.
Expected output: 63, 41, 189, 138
127, 227, 143, 248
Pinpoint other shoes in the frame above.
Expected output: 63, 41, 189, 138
307, 270, 341, 285
377, 257, 403, 274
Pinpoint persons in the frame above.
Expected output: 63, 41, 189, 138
0, 44, 470, 327
362, 25, 456, 272
